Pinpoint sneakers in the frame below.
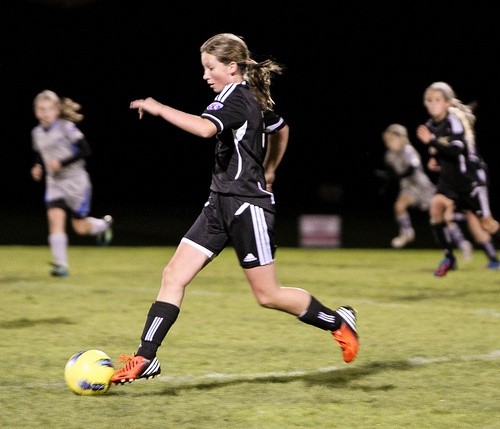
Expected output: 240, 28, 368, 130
110, 354, 161, 384
49, 263, 70, 277
98, 215, 113, 246
332, 305, 360, 364
435, 257, 457, 276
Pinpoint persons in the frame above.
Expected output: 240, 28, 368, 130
382, 80, 500, 277
31, 90, 113, 278
109, 33, 360, 386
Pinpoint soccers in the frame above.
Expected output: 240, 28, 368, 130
64, 349, 115, 395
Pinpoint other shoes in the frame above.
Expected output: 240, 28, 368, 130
392, 228, 415, 247
